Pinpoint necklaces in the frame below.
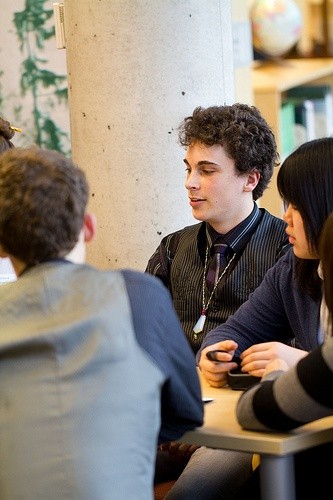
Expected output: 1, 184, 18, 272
193, 246, 237, 333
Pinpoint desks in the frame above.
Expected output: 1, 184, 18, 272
175, 366, 333, 500
254, 58, 333, 221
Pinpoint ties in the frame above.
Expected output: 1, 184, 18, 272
206, 237, 229, 292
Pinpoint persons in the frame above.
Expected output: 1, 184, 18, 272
195, 136, 333, 434
0, 148, 205, 500
1, 117, 86, 288
142, 103, 294, 368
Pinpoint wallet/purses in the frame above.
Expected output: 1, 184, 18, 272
206, 350, 262, 391
